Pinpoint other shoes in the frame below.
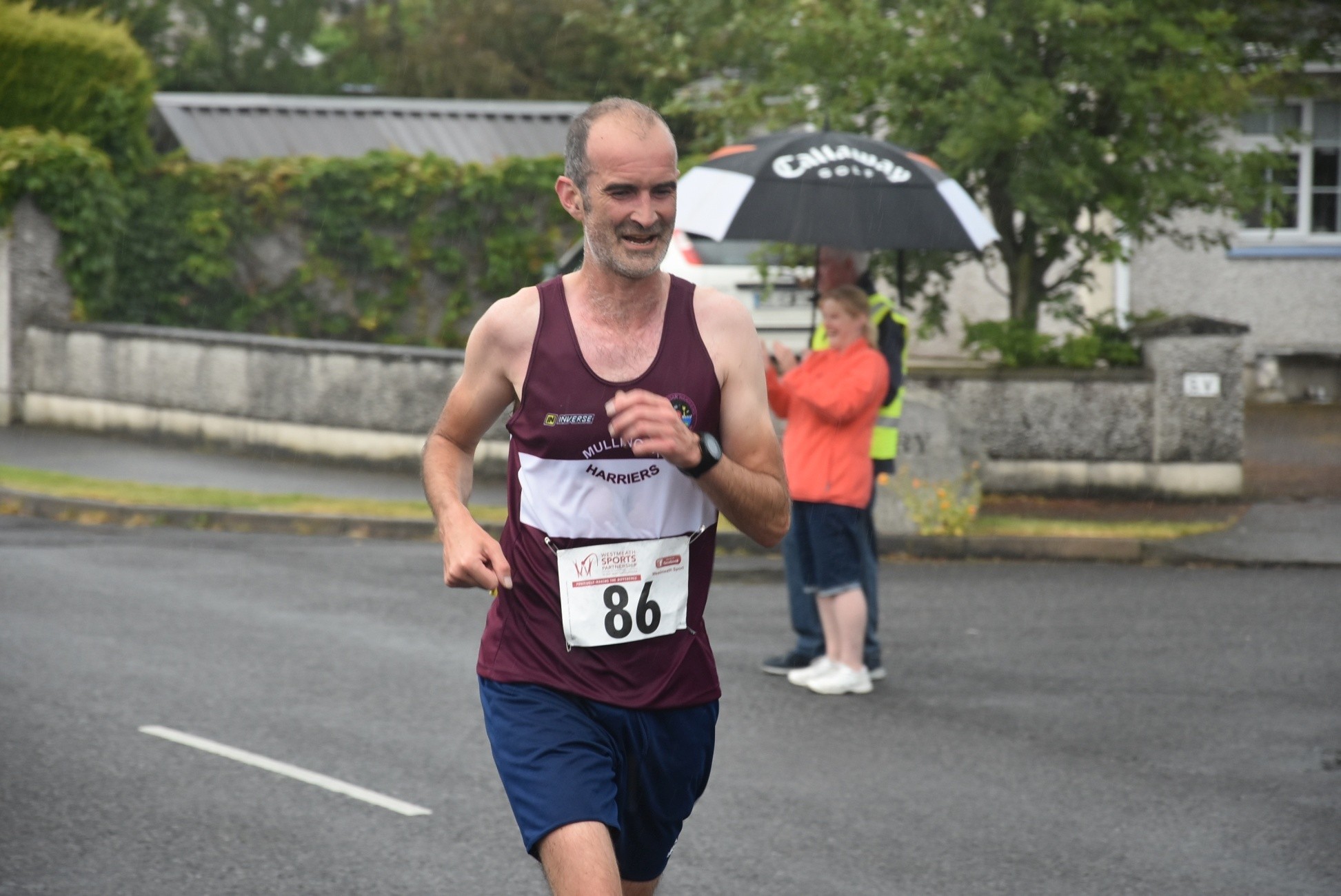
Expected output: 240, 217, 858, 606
788, 656, 872, 695
759, 651, 885, 680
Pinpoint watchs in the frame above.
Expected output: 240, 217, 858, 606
677, 432, 722, 478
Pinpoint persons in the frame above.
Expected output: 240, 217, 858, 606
762, 232, 912, 680
414, 94, 788, 896
760, 284, 876, 695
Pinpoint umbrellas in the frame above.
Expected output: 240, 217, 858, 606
673, 132, 1002, 359
